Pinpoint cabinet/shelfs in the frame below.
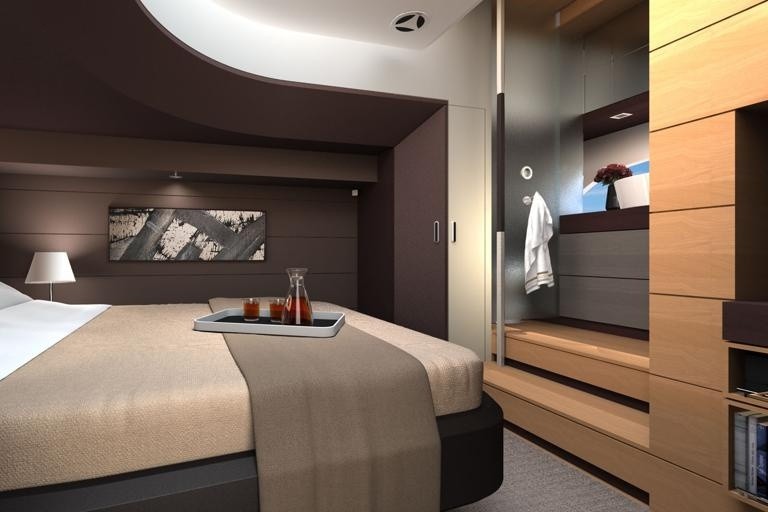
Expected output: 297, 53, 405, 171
726, 342, 767, 511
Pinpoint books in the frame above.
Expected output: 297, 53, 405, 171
732, 409, 768, 498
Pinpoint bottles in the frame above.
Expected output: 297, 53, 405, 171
284, 268, 314, 325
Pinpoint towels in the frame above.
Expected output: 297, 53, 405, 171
522, 191, 554, 296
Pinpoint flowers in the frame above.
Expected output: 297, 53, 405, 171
593, 163, 631, 186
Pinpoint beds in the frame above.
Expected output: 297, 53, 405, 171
1, 281, 505, 510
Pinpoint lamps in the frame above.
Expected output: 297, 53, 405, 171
26, 250, 76, 302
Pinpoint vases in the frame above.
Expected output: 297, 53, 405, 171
604, 175, 619, 207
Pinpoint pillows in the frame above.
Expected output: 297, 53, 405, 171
0, 281, 31, 309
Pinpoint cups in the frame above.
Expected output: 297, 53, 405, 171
269, 297, 285, 323
241, 297, 260, 322
